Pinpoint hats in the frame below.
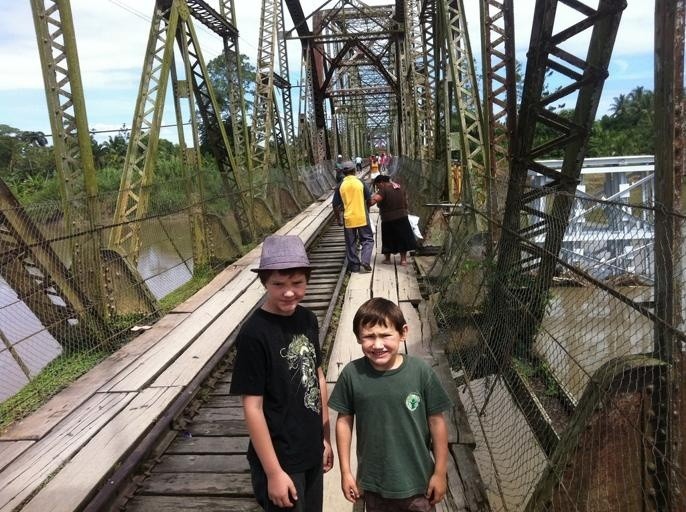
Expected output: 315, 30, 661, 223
249, 234, 321, 273
337, 155, 343, 159
342, 161, 356, 173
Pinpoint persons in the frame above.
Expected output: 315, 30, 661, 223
228, 234, 334, 512
370, 174, 419, 266
332, 152, 392, 183
331, 161, 375, 273
326, 296, 452, 512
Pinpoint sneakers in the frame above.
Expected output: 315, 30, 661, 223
381, 259, 392, 265
360, 261, 372, 272
400, 260, 407, 266
